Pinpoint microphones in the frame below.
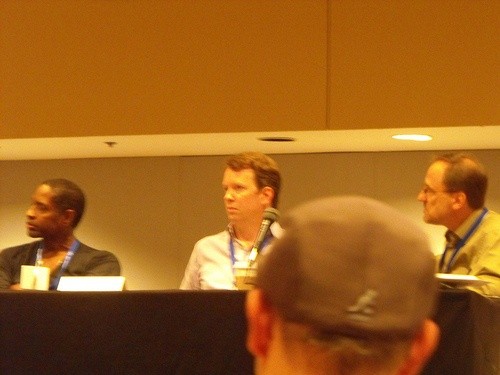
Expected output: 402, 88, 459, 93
249, 208, 280, 267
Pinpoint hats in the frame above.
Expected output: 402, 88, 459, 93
260, 195, 437, 340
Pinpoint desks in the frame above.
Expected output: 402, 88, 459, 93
0, 289, 500, 375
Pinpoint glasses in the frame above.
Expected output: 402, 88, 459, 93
422, 182, 457, 202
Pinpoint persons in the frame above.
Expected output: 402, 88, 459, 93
416, 151, 500, 299
0, 178, 121, 290
244, 195, 441, 375
178, 151, 283, 290
